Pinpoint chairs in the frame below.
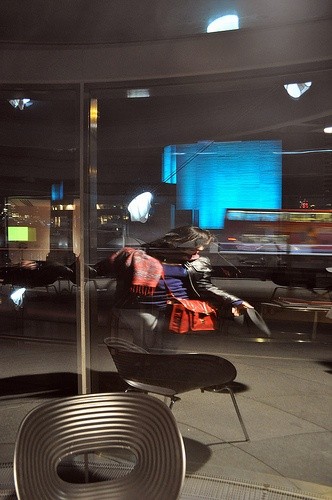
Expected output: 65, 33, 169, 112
13, 392, 187, 500
0, 250, 332, 335
104, 336, 250, 441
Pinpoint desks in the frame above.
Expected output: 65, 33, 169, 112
200, 253, 332, 280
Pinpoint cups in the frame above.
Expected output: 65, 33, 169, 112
302, 201, 308, 209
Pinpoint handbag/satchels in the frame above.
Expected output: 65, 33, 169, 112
168, 296, 220, 339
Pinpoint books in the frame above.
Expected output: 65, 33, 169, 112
272, 299, 309, 307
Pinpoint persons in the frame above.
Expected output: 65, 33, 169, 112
63, 223, 255, 355
